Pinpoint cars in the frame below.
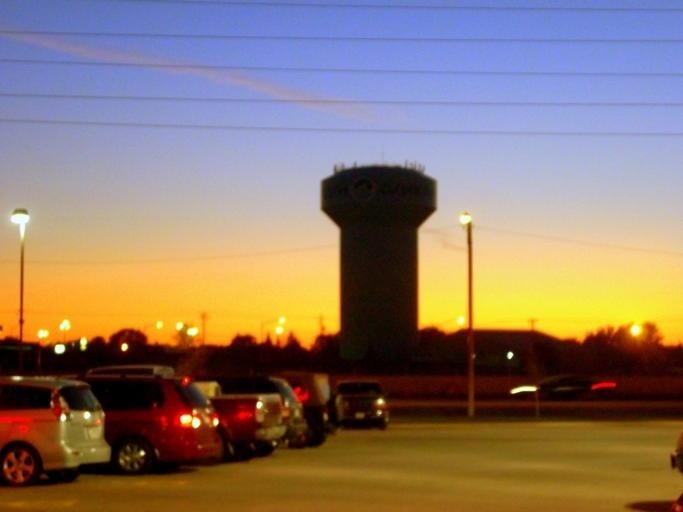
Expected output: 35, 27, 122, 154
60, 372, 226, 476
0, 374, 112, 481
330, 378, 390, 430
510, 376, 618, 400
272, 372, 335, 449
193, 373, 308, 457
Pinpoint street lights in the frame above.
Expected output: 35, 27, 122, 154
57, 320, 71, 345
457, 210, 477, 419
11, 206, 36, 370
199, 313, 210, 345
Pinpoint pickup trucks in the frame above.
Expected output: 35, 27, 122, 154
86, 364, 287, 459
